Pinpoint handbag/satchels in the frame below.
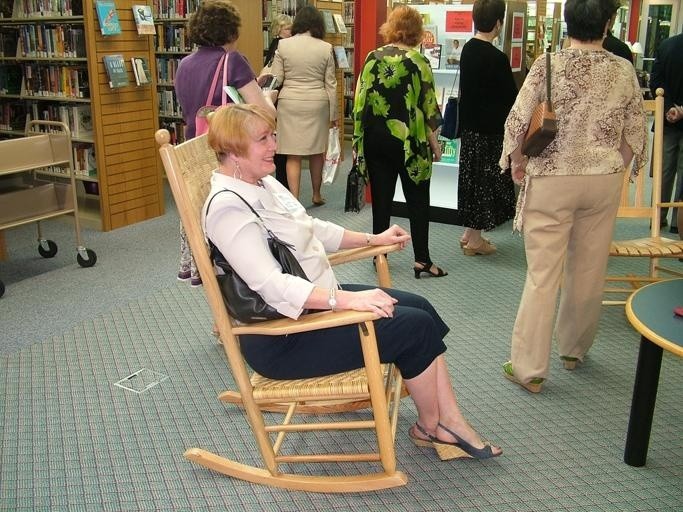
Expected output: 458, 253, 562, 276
205, 189, 313, 324
521, 53, 557, 159
343, 165, 367, 214
321, 127, 341, 185
440, 63, 461, 140
195, 52, 234, 138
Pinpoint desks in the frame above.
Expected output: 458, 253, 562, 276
623, 277, 683, 467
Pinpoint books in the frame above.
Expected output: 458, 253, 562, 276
0, 0, 97, 178
95, 0, 122, 36
132, 5, 157, 35
103, 55, 129, 88
151, 0, 200, 144
131, 57, 152, 86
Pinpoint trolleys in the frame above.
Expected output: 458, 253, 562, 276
0, 119, 96, 298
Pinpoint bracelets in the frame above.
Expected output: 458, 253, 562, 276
365, 233, 370, 245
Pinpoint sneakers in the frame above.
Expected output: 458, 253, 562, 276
191, 278, 203, 288
649, 219, 679, 234
177, 270, 192, 281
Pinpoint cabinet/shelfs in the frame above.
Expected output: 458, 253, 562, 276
389, 69, 460, 227
1, 1, 165, 230
154, 1, 356, 181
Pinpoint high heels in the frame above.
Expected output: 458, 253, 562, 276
407, 421, 435, 449
463, 239, 497, 256
431, 421, 503, 461
372, 256, 448, 279
503, 357, 545, 394
559, 355, 579, 371
459, 236, 490, 249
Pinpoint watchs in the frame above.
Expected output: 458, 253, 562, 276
328, 288, 336, 312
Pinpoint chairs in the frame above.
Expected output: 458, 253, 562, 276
559, 86, 683, 306
155, 111, 411, 493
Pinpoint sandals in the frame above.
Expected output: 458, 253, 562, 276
310, 197, 327, 207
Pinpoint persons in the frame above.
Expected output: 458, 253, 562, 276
650, 25, 683, 233
174, 1, 279, 287
597, 1, 633, 64
351, 7, 448, 279
201, 102, 502, 462
498, 1, 649, 394
264, 14, 292, 65
270, 5, 340, 204
459, 0, 519, 256
